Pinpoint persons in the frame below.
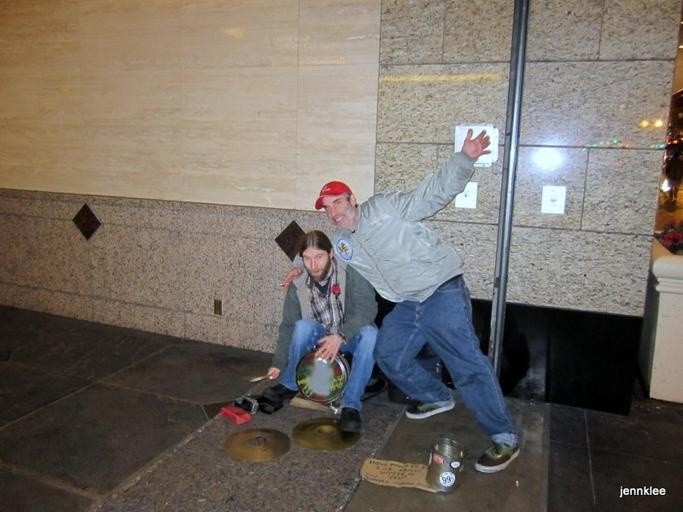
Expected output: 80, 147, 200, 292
666, 128, 683, 201
280, 129, 520, 474
262, 230, 378, 433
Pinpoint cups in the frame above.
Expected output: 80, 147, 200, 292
427, 438, 466, 491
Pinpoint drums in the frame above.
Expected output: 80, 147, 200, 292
296, 345, 351, 405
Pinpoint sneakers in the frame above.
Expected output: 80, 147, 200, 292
474, 442, 520, 473
405, 388, 455, 419
261, 383, 298, 402
340, 407, 362, 432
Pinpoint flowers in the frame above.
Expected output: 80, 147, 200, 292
658, 218, 683, 257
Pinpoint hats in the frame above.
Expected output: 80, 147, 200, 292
315, 181, 352, 210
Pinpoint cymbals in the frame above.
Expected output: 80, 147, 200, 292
292, 417, 360, 452
224, 428, 290, 462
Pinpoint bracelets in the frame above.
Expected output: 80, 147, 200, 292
336, 331, 346, 343
